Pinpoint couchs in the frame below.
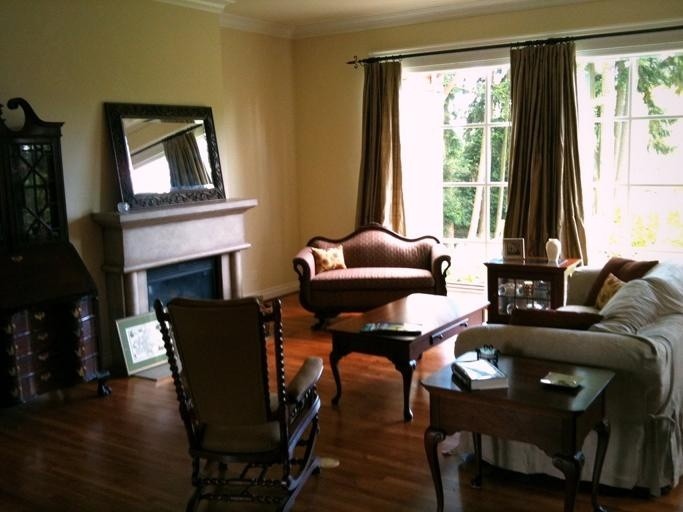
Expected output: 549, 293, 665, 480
292, 222, 452, 330
439, 260, 682, 498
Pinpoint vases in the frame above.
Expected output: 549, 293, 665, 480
544, 236, 561, 267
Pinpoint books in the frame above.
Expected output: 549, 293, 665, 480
449, 358, 509, 392
358, 321, 423, 336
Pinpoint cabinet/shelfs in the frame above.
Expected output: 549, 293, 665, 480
0, 94, 113, 407
484, 255, 584, 325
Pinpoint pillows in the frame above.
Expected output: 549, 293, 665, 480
509, 307, 604, 330
309, 244, 347, 273
581, 254, 657, 308
593, 272, 626, 310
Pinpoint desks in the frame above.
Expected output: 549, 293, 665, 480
416, 347, 618, 510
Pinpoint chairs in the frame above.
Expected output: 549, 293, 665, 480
152, 293, 326, 511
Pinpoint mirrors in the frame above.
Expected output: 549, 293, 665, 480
102, 97, 228, 216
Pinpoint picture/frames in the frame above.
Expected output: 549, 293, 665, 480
115, 312, 164, 377
502, 236, 525, 260
